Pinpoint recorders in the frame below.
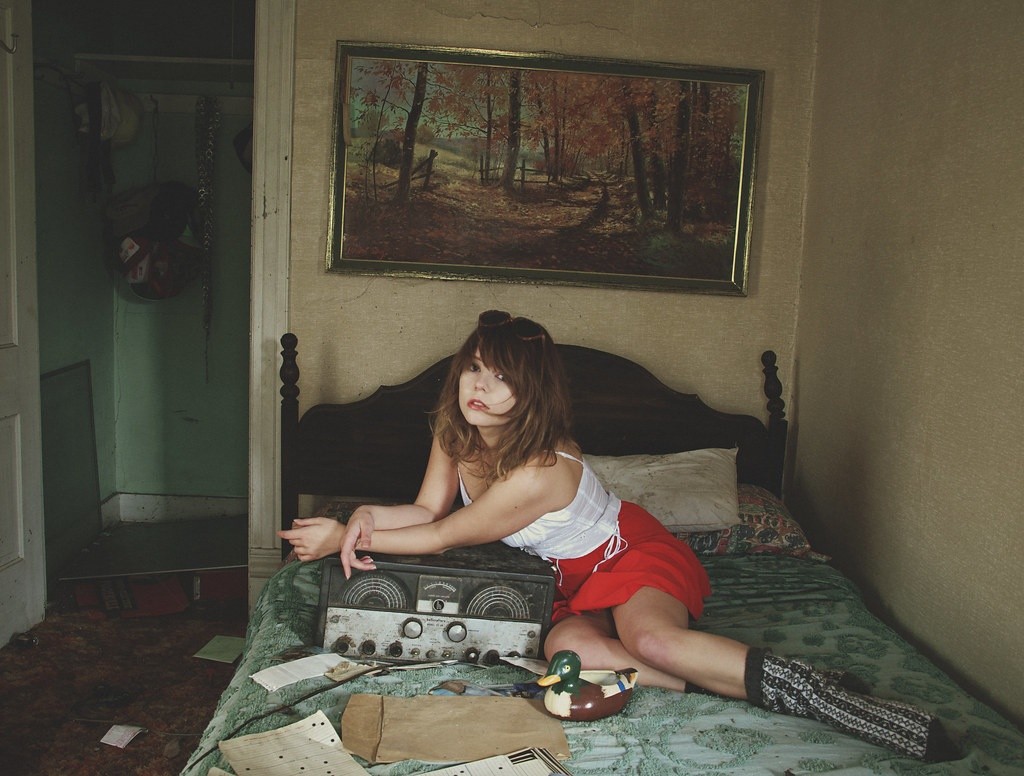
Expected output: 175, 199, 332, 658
315, 541, 557, 664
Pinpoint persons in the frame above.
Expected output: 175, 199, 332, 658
276, 317, 963, 762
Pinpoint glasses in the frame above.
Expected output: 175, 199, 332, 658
478, 309, 546, 390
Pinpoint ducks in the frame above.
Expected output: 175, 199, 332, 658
536, 650, 637, 721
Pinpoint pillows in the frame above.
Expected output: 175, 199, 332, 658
673, 484, 834, 556
580, 445, 740, 533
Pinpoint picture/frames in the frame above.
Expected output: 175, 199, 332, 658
322, 39, 765, 296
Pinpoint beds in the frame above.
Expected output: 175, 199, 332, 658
178, 332, 1024, 776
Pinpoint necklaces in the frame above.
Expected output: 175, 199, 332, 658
479, 452, 489, 490
